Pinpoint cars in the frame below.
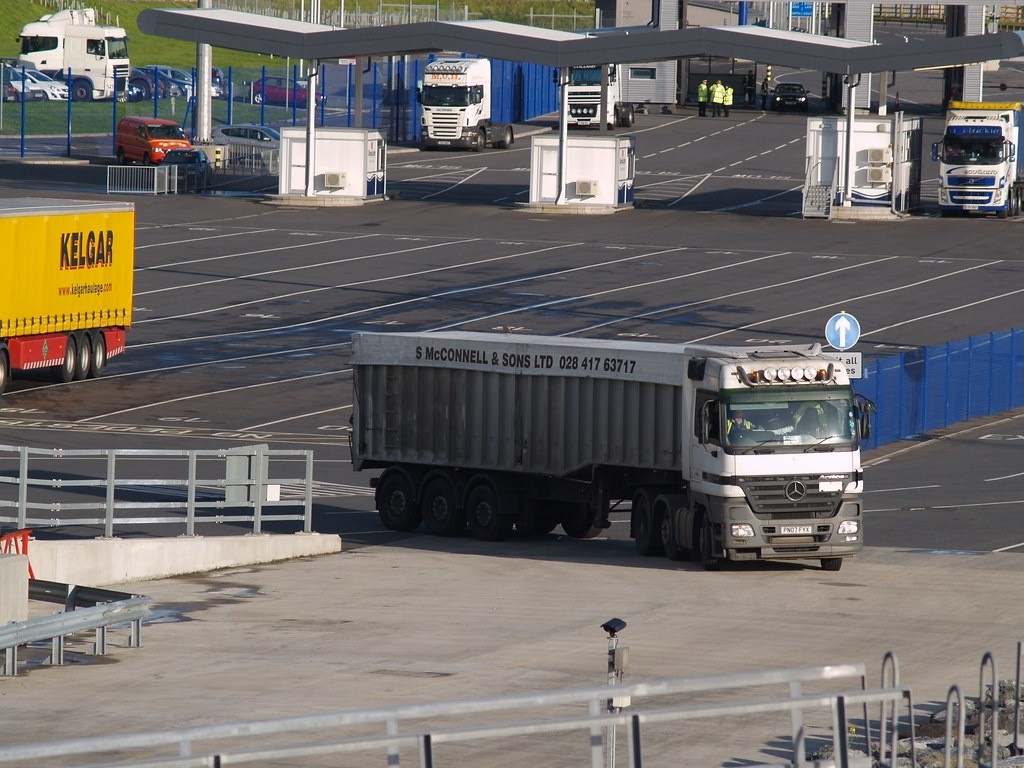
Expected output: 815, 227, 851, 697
129, 64, 228, 101
212, 125, 280, 167
158, 147, 212, 193
248, 76, 327, 108
771, 83, 811, 111
0, 63, 72, 102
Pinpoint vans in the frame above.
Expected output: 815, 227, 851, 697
116, 117, 192, 166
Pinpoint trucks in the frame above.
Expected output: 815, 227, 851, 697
553, 63, 635, 130
16, 8, 130, 102
417, 57, 514, 152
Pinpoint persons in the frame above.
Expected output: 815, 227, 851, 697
742, 70, 756, 107
709, 80, 726, 118
727, 410, 756, 435
761, 78, 768, 110
724, 84, 734, 118
698, 80, 707, 117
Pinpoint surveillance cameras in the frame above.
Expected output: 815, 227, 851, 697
600, 618, 627, 632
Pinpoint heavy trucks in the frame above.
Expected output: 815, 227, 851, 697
932, 99, 1024, 218
349, 332, 871, 571
1, 197, 135, 387
